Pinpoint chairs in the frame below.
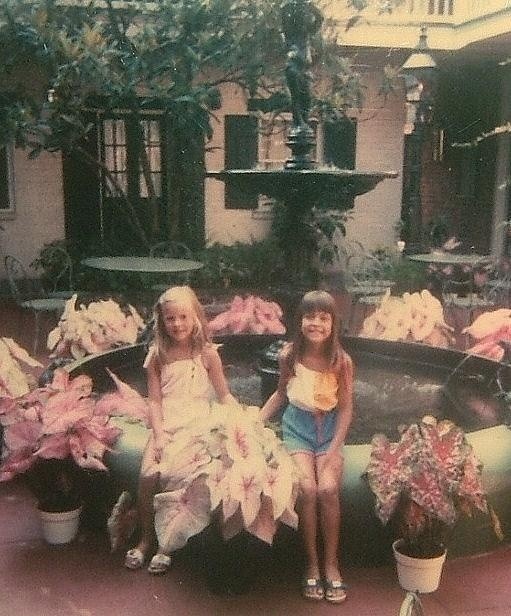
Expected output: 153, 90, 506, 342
149, 243, 194, 303
5, 245, 88, 356
335, 220, 509, 349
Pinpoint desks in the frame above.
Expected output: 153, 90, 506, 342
81, 255, 204, 312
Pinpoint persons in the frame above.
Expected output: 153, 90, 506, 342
259, 288, 355, 603
122, 286, 239, 575
278, 2, 326, 136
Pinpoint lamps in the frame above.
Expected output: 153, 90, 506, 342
400, 24, 441, 73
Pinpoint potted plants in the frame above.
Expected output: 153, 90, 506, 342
368, 417, 503, 596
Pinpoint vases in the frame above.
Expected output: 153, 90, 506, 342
34, 500, 84, 542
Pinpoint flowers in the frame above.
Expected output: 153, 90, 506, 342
1, 297, 149, 506
209, 295, 288, 336
458, 307, 511, 366
147, 395, 300, 559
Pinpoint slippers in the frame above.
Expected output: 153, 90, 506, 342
325, 577, 347, 602
125, 549, 144, 569
147, 553, 172, 573
301, 574, 325, 600
